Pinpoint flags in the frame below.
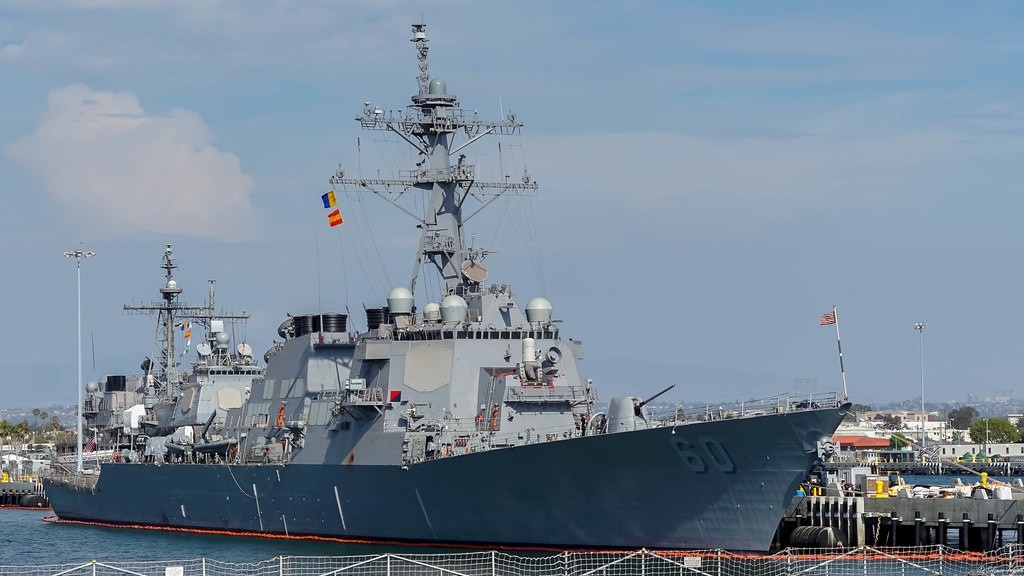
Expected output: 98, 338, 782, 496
85, 430, 95, 453
328, 210, 343, 228
175, 321, 192, 357
321, 191, 337, 208
819, 311, 836, 325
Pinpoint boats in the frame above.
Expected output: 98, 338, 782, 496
40, 26, 853, 553
